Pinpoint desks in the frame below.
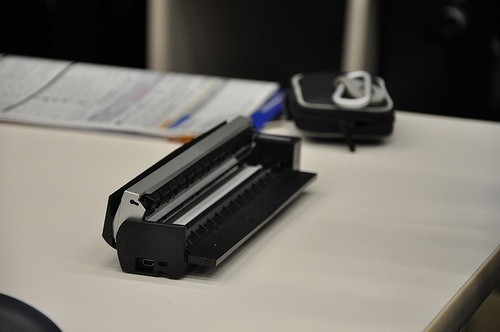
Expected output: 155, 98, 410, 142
1, 111, 500, 331
